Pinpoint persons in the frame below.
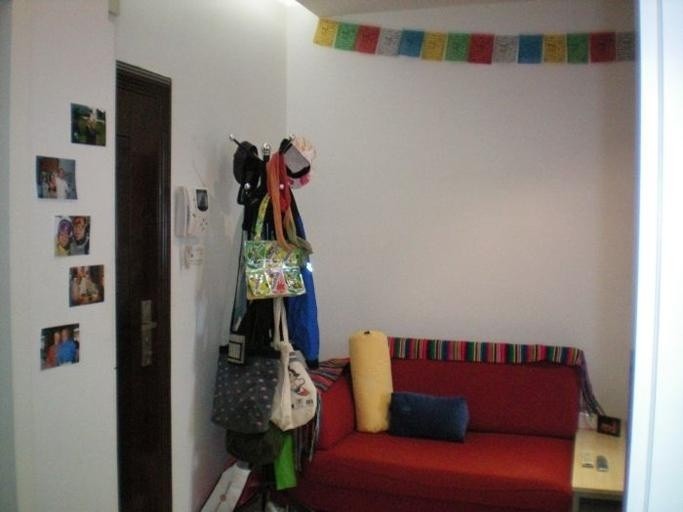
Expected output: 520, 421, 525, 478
46, 167, 70, 199
44, 331, 60, 367
53, 327, 75, 365
55, 218, 74, 256
68, 265, 103, 305
85, 111, 98, 145
70, 110, 81, 143
68, 216, 89, 255
47, 174, 58, 198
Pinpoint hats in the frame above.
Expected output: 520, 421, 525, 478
233, 141, 257, 183
279, 136, 315, 190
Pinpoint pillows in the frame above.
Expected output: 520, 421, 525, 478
349, 326, 469, 443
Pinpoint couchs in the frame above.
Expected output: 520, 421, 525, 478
284, 337, 587, 511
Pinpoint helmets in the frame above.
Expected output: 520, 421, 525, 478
58, 216, 87, 238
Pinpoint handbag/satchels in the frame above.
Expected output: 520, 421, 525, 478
242, 193, 306, 299
211, 343, 282, 435
268, 339, 318, 432
199, 426, 304, 511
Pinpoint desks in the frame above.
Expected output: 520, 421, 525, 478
568, 411, 626, 510
